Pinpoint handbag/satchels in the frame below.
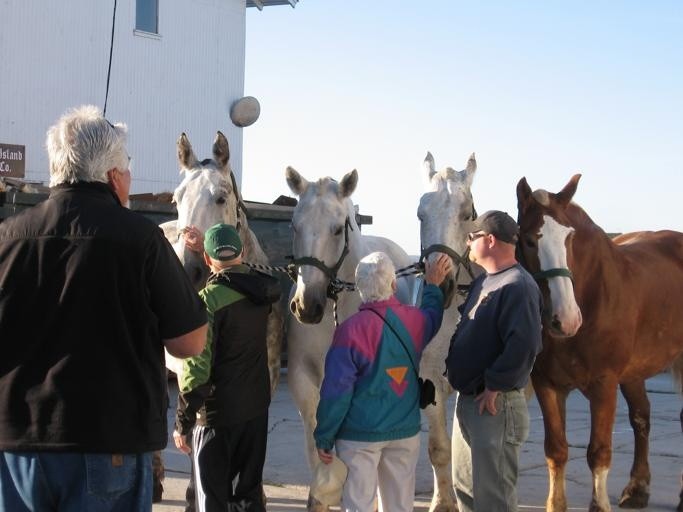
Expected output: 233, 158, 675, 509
416, 376, 436, 407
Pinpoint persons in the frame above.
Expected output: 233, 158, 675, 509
0, 104, 208, 512
173, 225, 282, 512
313, 250, 454, 512
442, 210, 543, 512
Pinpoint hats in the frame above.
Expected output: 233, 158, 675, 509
309, 455, 348, 508
204, 222, 242, 261
466, 211, 520, 246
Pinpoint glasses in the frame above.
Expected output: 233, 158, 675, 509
467, 231, 488, 241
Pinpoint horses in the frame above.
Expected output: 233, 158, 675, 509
152, 131, 285, 512
414, 150, 533, 512
516, 174, 683, 512
285, 166, 414, 512
